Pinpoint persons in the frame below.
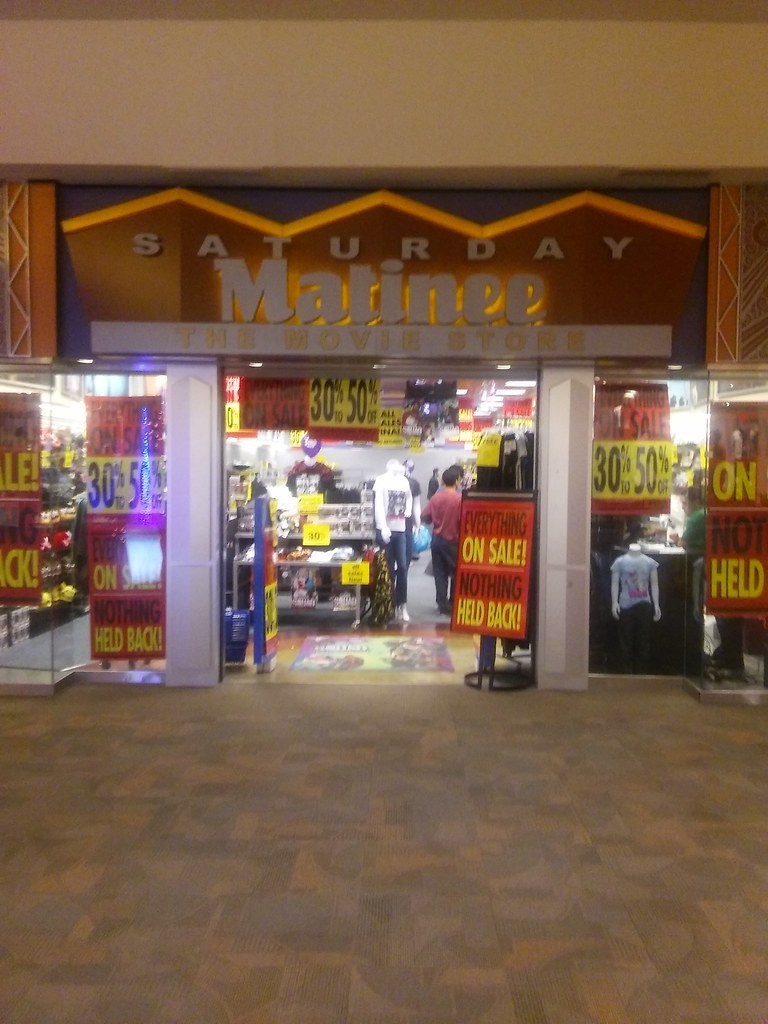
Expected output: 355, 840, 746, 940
371, 463, 413, 622
419, 465, 465, 612
668, 487, 744, 669
288, 454, 334, 493
407, 477, 421, 564
251, 473, 266, 500
610, 544, 662, 674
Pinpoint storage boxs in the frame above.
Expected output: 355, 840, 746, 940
0, 605, 26, 650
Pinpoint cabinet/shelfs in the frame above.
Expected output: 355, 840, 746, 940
234, 531, 376, 631
29, 466, 77, 638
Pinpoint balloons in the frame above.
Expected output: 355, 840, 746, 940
301, 434, 322, 458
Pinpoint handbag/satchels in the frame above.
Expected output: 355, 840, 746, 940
413, 526, 432, 552
